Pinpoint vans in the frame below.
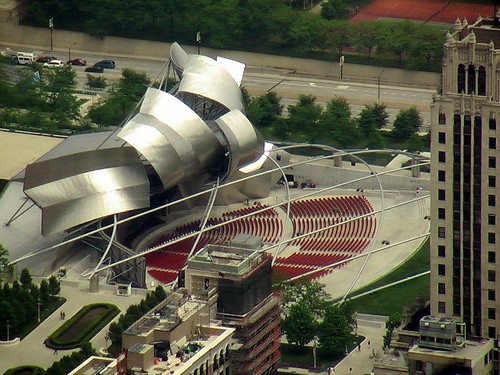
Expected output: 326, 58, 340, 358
11, 51, 38, 66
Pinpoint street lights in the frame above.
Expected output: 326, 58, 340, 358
377, 70, 385, 104
69, 42, 76, 60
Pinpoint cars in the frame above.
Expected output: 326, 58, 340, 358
66, 58, 86, 66
95, 60, 115, 69
43, 60, 65, 69
85, 65, 104, 73
35, 56, 59, 62
